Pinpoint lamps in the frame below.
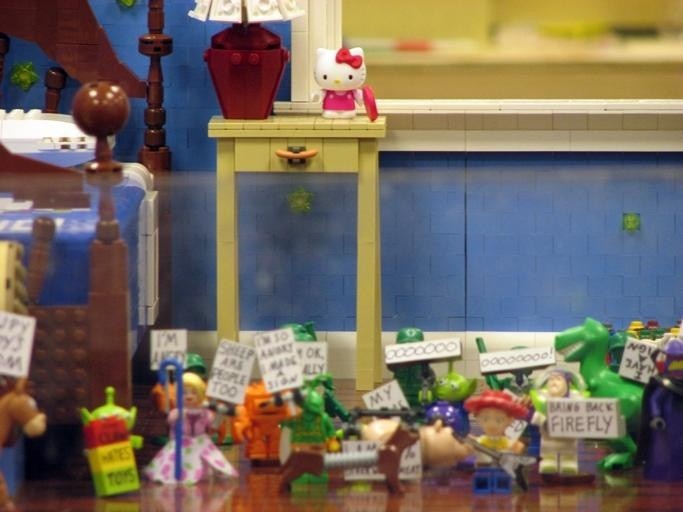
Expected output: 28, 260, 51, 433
187, 0, 306, 121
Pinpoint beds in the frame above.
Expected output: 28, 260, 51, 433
1, 0, 176, 427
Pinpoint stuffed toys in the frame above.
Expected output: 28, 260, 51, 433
313, 45, 379, 122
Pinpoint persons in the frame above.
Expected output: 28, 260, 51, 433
144, 370, 246, 485
533, 369, 590, 477
0, 307, 683, 512
463, 388, 533, 497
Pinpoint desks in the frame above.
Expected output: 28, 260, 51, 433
204, 114, 388, 393
